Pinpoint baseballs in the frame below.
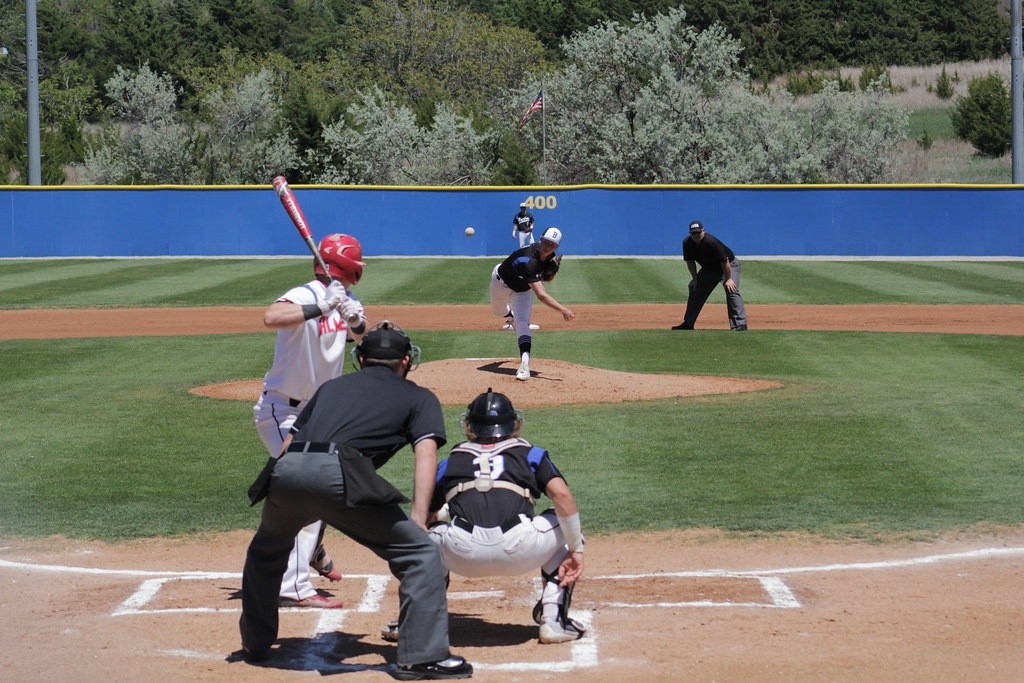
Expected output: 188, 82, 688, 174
465, 227, 474, 236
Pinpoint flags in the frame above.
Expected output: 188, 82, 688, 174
519, 89, 545, 127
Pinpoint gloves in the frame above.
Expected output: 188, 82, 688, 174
335, 296, 363, 329
316, 281, 347, 315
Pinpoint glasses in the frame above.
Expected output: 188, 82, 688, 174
542, 241, 555, 249
690, 232, 701, 236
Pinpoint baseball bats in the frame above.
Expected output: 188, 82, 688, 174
271, 175, 357, 323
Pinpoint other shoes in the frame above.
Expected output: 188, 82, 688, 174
672, 322, 694, 330
731, 325, 747, 331
502, 325, 514, 330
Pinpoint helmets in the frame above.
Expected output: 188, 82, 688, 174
467, 388, 518, 440
540, 227, 562, 246
314, 234, 366, 288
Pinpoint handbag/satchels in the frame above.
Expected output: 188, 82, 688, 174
247, 455, 276, 507
336, 444, 412, 507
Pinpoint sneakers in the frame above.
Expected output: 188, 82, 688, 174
393, 651, 473, 680
278, 594, 342, 608
381, 621, 398, 640
515, 364, 530, 380
309, 544, 342, 582
503, 311, 516, 332
246, 648, 271, 661
539, 615, 585, 644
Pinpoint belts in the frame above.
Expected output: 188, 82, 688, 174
496, 275, 506, 284
287, 442, 329, 453
454, 514, 533, 534
263, 391, 300, 407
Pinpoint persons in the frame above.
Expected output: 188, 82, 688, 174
382, 388, 587, 644
253, 233, 371, 608
237, 320, 473, 680
489, 227, 574, 379
671, 221, 747, 331
513, 202, 535, 248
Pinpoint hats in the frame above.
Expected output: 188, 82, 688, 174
361, 326, 410, 361
689, 221, 703, 232
520, 203, 526, 208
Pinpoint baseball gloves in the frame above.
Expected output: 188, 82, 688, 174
545, 254, 563, 275
